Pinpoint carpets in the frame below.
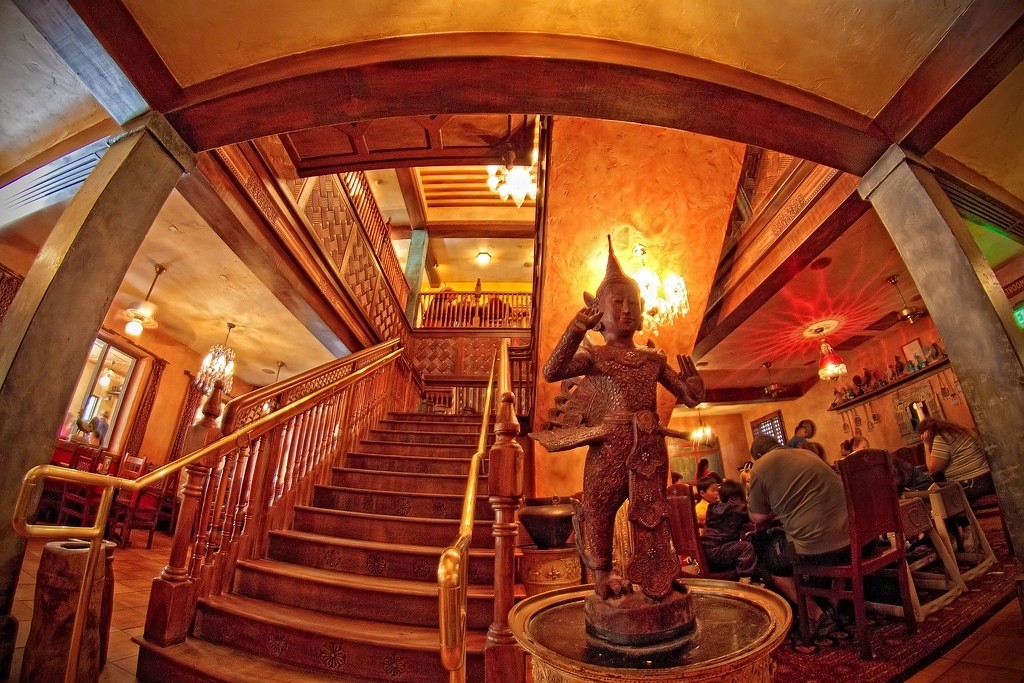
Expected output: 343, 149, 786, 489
769, 511, 1024, 683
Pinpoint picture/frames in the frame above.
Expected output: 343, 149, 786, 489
901, 338, 926, 367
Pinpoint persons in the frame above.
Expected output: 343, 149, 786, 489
670, 417, 994, 642
542, 233, 706, 600
60, 407, 109, 446
423, 287, 511, 327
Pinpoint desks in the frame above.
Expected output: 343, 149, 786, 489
739, 519, 782, 563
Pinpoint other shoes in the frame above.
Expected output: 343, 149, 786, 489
809, 613, 837, 638
952, 531, 981, 550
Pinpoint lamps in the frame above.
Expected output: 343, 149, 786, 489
630, 244, 690, 336
763, 361, 783, 397
195, 323, 236, 395
99, 361, 115, 387
802, 320, 848, 384
476, 253, 491, 266
500, 115, 518, 171
887, 275, 918, 324
487, 148, 539, 207
124, 264, 166, 337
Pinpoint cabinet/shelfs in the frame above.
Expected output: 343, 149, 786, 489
864, 480, 998, 621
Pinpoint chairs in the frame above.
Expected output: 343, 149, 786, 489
788, 448, 917, 660
892, 444, 926, 465
947, 493, 1016, 556
56, 443, 181, 550
666, 484, 740, 581
